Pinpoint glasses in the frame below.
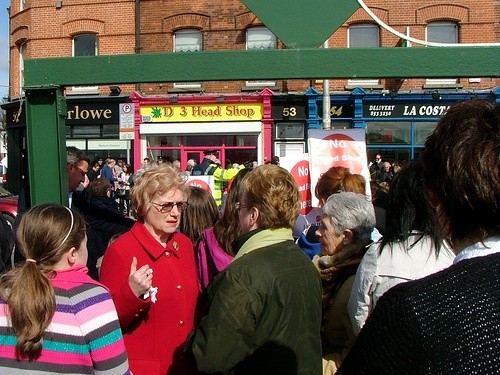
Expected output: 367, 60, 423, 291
149, 201, 184, 213
234, 201, 253, 210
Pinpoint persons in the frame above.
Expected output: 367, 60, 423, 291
332, 96, 500, 374
68, 144, 426, 285
63, 146, 137, 234
183, 162, 327, 374
346, 160, 457, 341
99, 162, 199, 375
310, 191, 380, 375
10, 144, 91, 268
1, 203, 130, 375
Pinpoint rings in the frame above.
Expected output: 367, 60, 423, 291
144, 273, 149, 277
